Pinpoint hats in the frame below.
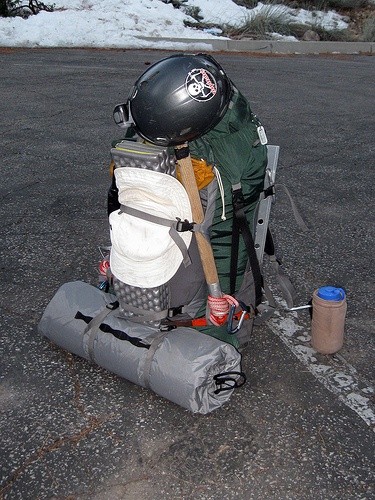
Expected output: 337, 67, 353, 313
108, 166, 193, 288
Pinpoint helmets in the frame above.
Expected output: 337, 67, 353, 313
112, 51, 236, 147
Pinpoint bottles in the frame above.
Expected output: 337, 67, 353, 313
312, 286, 347, 354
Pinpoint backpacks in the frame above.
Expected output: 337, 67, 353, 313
36, 74, 281, 414
